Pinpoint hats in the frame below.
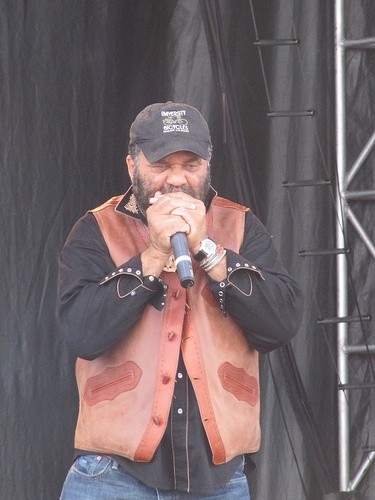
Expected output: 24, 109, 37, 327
129, 101, 213, 165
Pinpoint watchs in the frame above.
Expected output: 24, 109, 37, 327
193, 238, 216, 262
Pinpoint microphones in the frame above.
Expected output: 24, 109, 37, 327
170, 232, 194, 288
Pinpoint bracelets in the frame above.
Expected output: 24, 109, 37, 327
199, 245, 226, 272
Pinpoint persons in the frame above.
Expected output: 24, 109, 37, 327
56, 101, 305, 500
